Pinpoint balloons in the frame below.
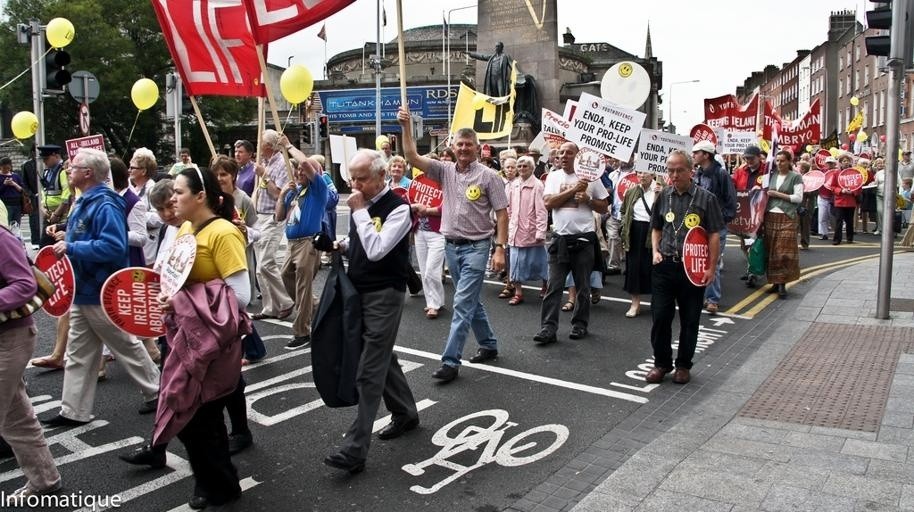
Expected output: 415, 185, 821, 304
46, 17, 75, 48
600, 60, 652, 111
131, 78, 159, 109
850, 96, 859, 105
280, 64, 313, 105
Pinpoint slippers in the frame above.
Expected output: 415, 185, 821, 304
32, 356, 63, 368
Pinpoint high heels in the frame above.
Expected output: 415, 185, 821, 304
626, 304, 640, 317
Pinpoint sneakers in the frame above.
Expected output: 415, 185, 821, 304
704, 299, 718, 312
284, 336, 311, 350
427, 310, 437, 318
740, 271, 755, 288
7, 484, 62, 505
569, 325, 588, 339
533, 328, 556, 342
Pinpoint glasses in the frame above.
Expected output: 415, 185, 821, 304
69, 165, 87, 170
518, 165, 528, 168
131, 166, 138, 170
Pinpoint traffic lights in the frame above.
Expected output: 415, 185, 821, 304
864, 1, 893, 56
45, 50, 72, 93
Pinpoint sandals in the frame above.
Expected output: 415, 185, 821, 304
501, 287, 600, 312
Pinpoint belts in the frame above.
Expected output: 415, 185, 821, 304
448, 238, 468, 245
666, 255, 682, 262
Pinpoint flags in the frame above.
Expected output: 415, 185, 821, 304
317, 23, 327, 42
444, 19, 448, 37
383, 8, 388, 26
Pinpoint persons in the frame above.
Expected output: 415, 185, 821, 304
460, 40, 519, 112
1, 105, 914, 512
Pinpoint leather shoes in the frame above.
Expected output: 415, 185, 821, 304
431, 365, 458, 379
39, 414, 89, 426
119, 445, 165, 468
646, 367, 666, 382
228, 430, 254, 454
251, 305, 294, 319
469, 348, 497, 363
378, 420, 419, 439
325, 452, 365, 472
674, 367, 690, 383
767, 282, 786, 296
188, 495, 207, 509
139, 400, 157, 414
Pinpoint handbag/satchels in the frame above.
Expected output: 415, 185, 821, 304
749, 222, 766, 276
21, 192, 34, 215
0, 257, 56, 324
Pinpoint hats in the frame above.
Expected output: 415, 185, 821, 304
528, 147, 543, 157
902, 150, 912, 155
825, 156, 837, 165
37, 145, 61, 155
741, 147, 760, 158
692, 141, 715, 154
